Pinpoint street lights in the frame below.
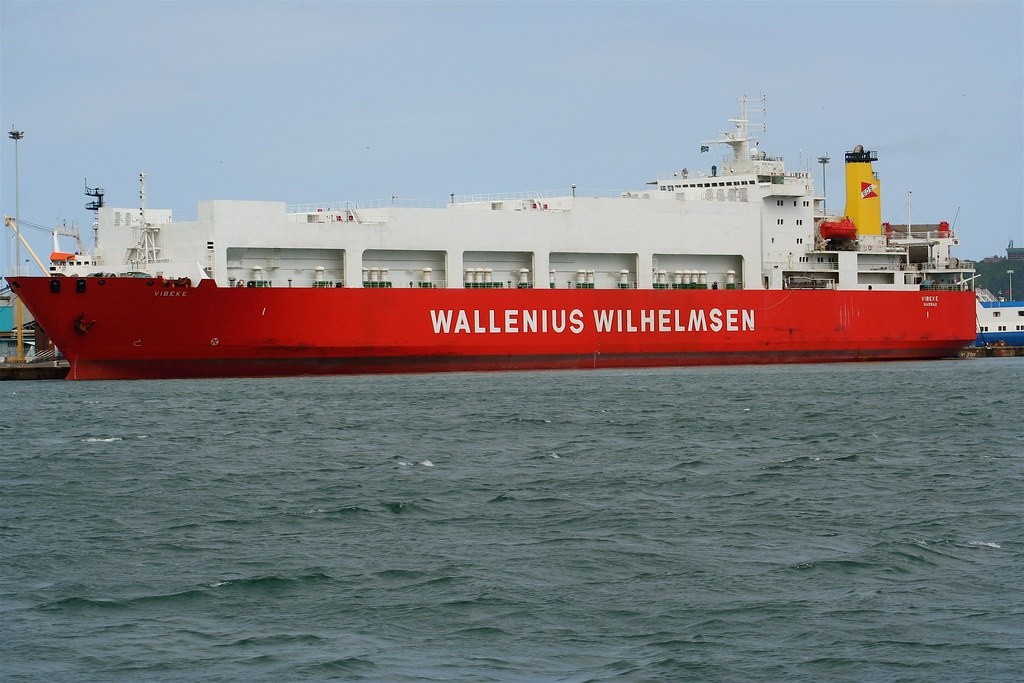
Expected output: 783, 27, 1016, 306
8, 122, 25, 274
817, 157, 831, 216
1007, 270, 1014, 302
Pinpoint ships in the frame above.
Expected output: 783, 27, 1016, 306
2, 94, 977, 380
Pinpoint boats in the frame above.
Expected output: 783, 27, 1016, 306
50, 251, 75, 262
969, 289, 1024, 347
820, 217, 857, 240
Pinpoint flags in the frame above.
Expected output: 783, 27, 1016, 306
701, 145, 709, 153
